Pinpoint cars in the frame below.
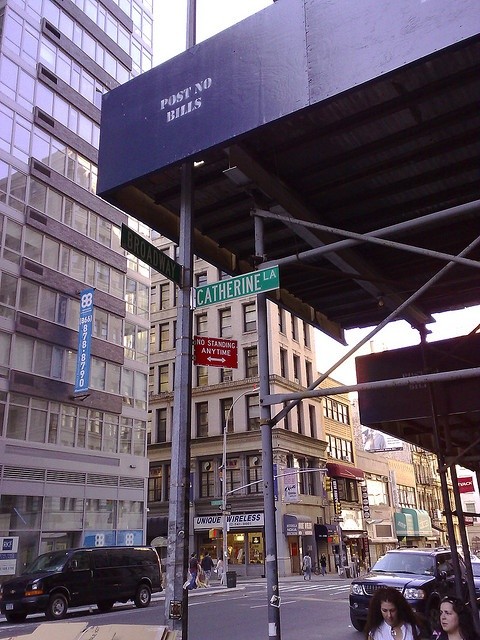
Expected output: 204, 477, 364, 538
468, 554, 480, 595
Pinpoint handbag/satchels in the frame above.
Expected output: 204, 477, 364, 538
301, 563, 306, 571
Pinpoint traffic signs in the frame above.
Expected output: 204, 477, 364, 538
196, 265, 280, 306
194, 335, 238, 369
121, 223, 180, 285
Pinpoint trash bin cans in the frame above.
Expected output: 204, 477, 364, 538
226, 571, 236, 587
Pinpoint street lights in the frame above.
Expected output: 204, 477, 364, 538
221, 388, 260, 585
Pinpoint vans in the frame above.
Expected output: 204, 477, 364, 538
1, 546, 163, 621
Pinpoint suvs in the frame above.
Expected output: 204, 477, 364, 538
350, 547, 473, 631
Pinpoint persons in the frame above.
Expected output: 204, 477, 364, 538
302, 552, 312, 580
187, 553, 201, 590
214, 558, 224, 580
319, 553, 327, 575
200, 551, 214, 587
362, 587, 423, 640
426, 596, 480, 640
